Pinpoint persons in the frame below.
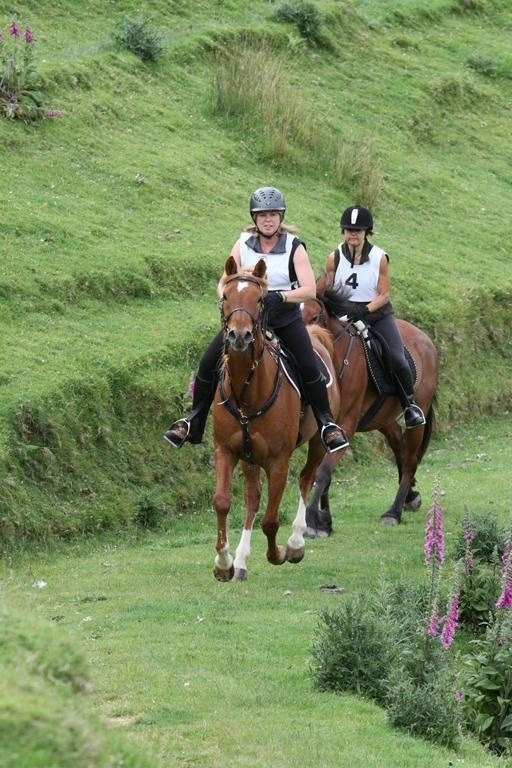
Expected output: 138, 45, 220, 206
165, 185, 347, 451
314, 201, 425, 428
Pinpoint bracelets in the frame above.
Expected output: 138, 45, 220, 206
279, 290, 288, 303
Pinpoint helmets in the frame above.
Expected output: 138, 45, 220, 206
340, 205, 373, 230
250, 187, 286, 212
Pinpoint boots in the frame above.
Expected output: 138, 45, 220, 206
163, 376, 219, 448
392, 366, 424, 427
305, 372, 346, 450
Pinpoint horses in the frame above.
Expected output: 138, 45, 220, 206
303, 269, 439, 538
209, 255, 343, 584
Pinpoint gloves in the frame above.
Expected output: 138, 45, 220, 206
263, 291, 284, 308
347, 304, 370, 323
